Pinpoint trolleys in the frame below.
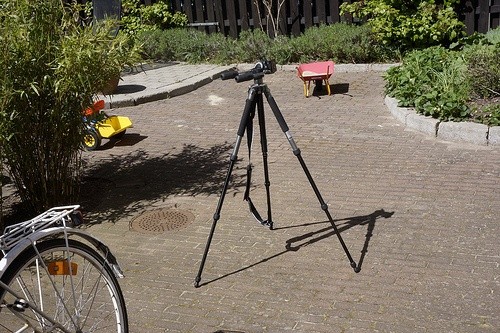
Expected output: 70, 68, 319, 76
295, 60, 334, 98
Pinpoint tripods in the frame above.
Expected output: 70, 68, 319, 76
194, 65, 359, 288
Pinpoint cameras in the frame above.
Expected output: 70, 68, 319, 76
262, 60, 277, 75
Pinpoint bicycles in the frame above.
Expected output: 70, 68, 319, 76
0, 202, 129, 333
